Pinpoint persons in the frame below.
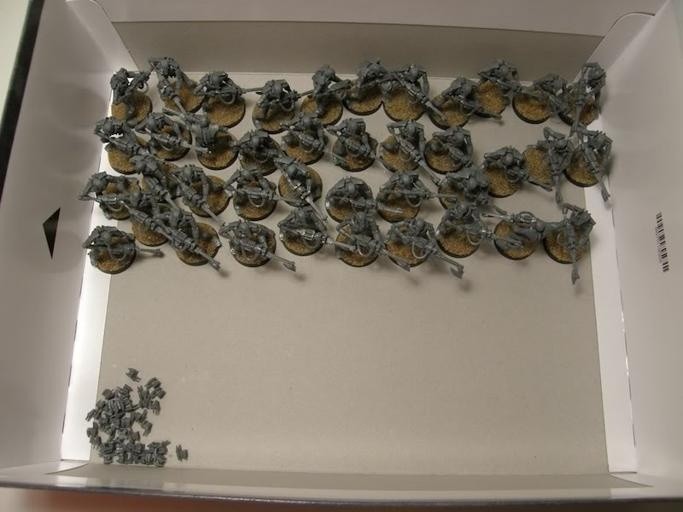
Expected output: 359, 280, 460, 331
76, 55, 613, 284
76, 55, 614, 285
